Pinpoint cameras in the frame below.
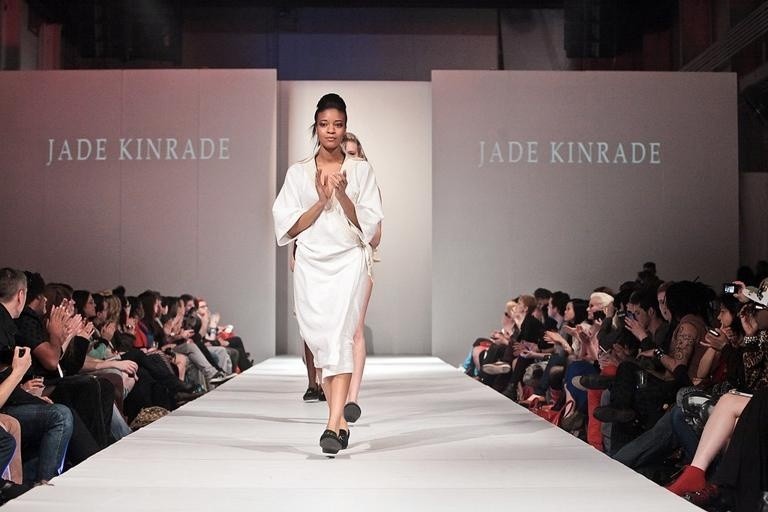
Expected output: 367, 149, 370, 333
593, 309, 605, 320
721, 282, 738, 295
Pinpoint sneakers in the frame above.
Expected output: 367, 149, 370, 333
303, 383, 326, 402
343, 403, 361, 422
339, 429, 350, 449
593, 407, 635, 423
320, 430, 340, 453
482, 362, 511, 374
210, 371, 237, 384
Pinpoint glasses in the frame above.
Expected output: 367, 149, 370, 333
752, 304, 768, 314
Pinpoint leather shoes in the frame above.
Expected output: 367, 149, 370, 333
167, 383, 204, 406
572, 376, 610, 392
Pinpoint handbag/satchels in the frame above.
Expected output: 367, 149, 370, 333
128, 407, 172, 430
529, 398, 573, 426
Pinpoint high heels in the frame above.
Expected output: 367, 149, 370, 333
518, 395, 545, 410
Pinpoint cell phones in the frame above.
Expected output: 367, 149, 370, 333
709, 329, 719, 337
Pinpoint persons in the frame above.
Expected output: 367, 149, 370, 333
463, 256, 767, 511
1, 265, 253, 503
289, 237, 329, 403
275, 90, 382, 458
340, 134, 382, 421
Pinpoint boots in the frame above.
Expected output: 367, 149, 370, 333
666, 465, 705, 495
549, 386, 565, 410
502, 382, 517, 400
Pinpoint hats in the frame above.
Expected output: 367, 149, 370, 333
743, 277, 768, 308
